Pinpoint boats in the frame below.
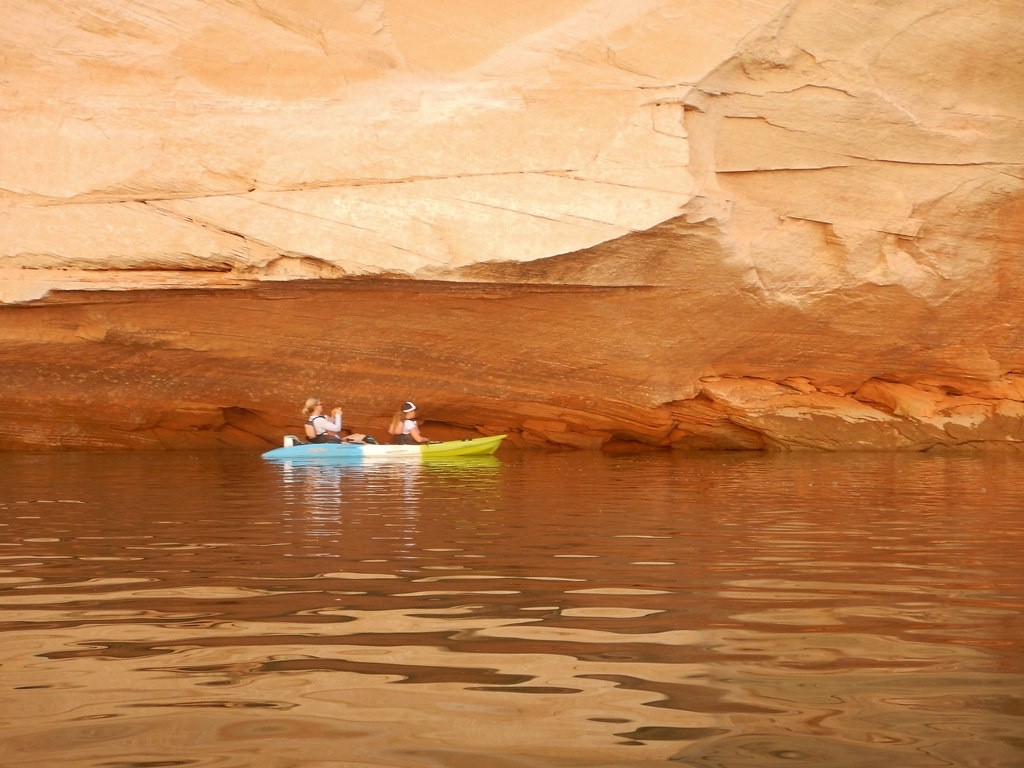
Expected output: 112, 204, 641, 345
260, 435, 507, 460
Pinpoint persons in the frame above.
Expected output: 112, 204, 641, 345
302, 398, 342, 443
389, 402, 429, 445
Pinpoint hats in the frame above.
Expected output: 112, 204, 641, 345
401, 402, 416, 412
